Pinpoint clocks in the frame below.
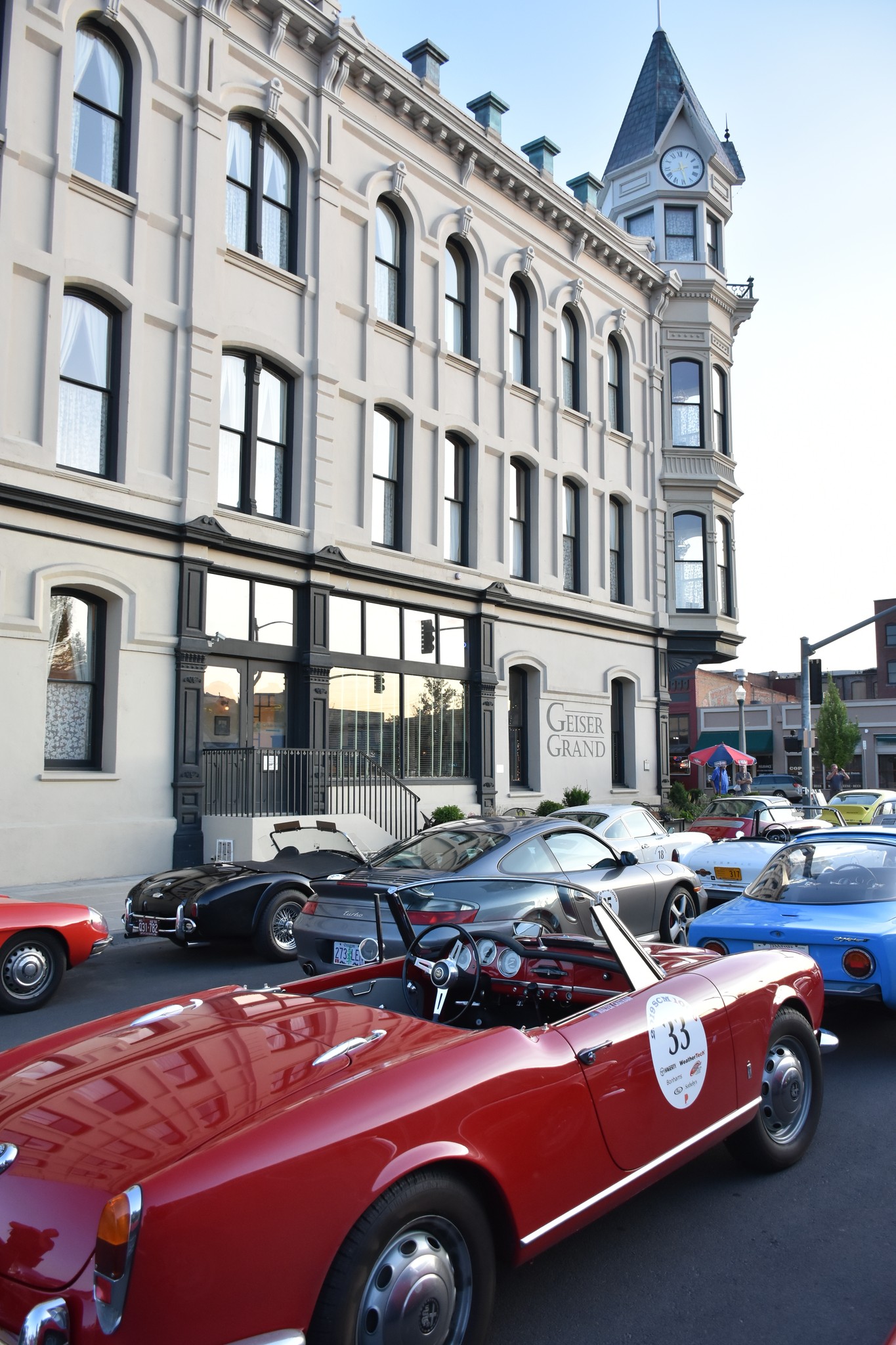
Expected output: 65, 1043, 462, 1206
659, 145, 706, 189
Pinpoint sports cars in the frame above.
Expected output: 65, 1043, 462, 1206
0, 875, 840, 1345
681, 806, 888, 899
686, 795, 833, 843
291, 816, 708, 977
121, 820, 432, 963
546, 803, 712, 862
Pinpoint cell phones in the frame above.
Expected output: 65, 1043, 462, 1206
837, 768, 842, 771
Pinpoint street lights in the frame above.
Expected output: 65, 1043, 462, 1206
735, 682, 747, 773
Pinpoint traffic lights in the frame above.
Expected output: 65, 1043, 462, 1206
374, 675, 385, 694
423, 623, 434, 650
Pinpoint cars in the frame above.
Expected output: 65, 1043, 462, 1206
685, 826, 896, 1049
813, 789, 896, 826
0, 894, 114, 1015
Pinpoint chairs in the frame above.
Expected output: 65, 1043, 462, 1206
739, 836, 769, 840
864, 884, 896, 899
796, 883, 824, 897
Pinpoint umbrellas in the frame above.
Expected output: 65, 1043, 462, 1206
688, 742, 757, 796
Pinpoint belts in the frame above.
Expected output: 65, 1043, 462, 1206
830, 789, 842, 791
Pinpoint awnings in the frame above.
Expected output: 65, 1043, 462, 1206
694, 731, 773, 754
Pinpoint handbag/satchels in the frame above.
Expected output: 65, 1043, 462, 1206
733, 784, 742, 791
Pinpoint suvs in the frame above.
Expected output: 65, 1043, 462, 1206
727, 773, 802, 804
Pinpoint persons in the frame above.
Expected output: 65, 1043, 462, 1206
710, 765, 729, 795
826, 764, 850, 801
735, 765, 752, 796
